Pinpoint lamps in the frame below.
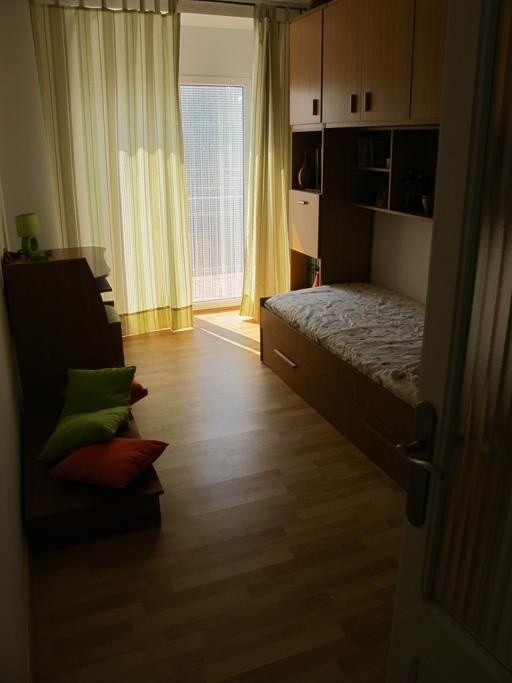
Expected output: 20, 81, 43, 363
14, 214, 40, 254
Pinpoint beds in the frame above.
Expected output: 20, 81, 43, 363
260, 283, 425, 528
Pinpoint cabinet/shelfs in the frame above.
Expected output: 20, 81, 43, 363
291, 125, 440, 291
287, 1, 448, 123
3, 248, 125, 407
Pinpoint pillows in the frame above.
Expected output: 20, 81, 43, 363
41, 366, 168, 486
